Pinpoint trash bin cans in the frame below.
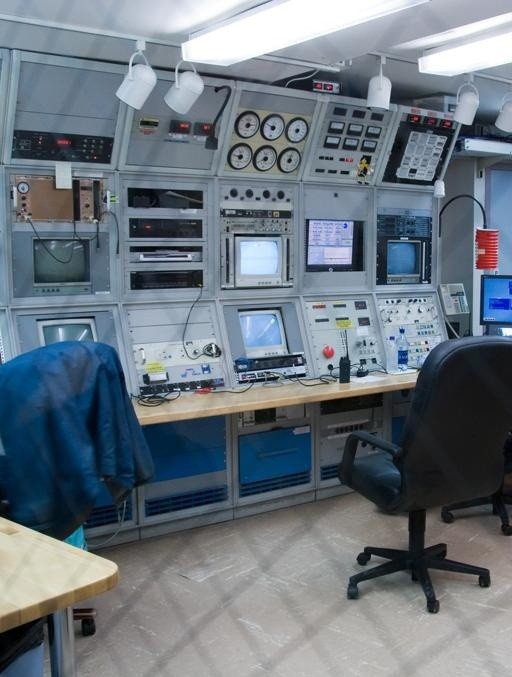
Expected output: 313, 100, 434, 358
0, 618, 45, 676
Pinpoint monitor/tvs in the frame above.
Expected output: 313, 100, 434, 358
386, 240, 421, 284
235, 237, 282, 287
31, 236, 92, 297
239, 310, 289, 359
306, 219, 359, 272
480, 275, 512, 325
37, 318, 98, 348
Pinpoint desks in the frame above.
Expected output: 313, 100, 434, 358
0, 517, 119, 677
128, 367, 423, 427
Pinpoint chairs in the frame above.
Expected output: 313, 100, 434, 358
334, 337, 512, 614
441, 435, 512, 537
0, 342, 153, 636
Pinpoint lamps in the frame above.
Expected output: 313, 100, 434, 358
162, 59, 207, 117
433, 179, 446, 199
439, 195, 499, 269
181, 0, 427, 66
417, 21, 512, 77
366, 51, 512, 134
116, 40, 158, 106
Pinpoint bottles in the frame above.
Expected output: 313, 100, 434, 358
395, 328, 409, 371
